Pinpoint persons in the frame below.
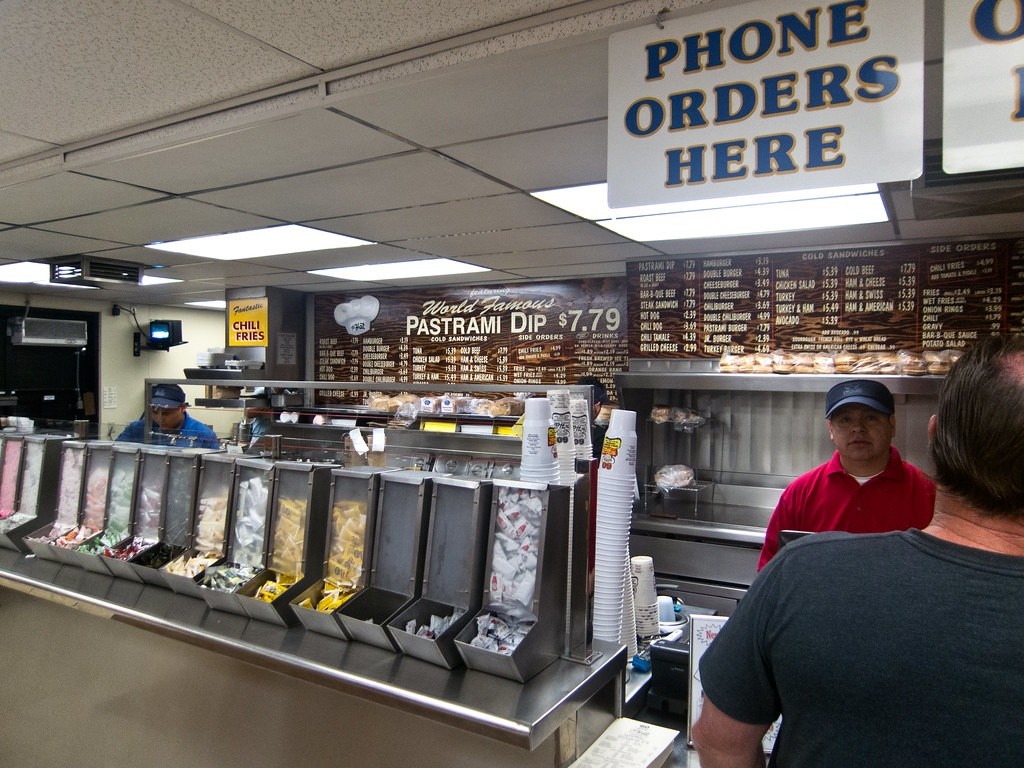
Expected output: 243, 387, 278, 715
692, 334, 1024, 768
757, 380, 936, 572
575, 375, 607, 468
244, 362, 270, 450
113, 384, 220, 449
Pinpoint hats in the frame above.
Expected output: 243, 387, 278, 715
149, 386, 185, 408
826, 380, 895, 416
574, 377, 611, 403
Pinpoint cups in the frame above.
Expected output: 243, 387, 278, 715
1, 416, 34, 434
235, 423, 250, 442
73, 420, 89, 438
518, 390, 675, 663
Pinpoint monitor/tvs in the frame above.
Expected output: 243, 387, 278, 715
149, 319, 182, 346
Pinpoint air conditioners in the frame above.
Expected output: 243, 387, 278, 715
6, 317, 88, 347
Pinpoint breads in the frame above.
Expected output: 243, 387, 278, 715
718, 353, 965, 375
649, 407, 703, 425
595, 405, 620, 424
367, 394, 525, 416
